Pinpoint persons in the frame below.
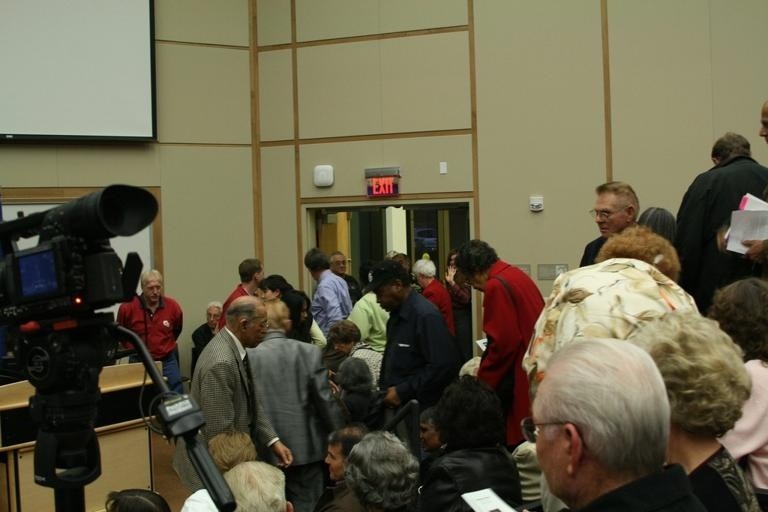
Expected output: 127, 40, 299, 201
104, 489, 171, 512
517, 98, 768, 512
175, 239, 545, 512
117, 269, 185, 395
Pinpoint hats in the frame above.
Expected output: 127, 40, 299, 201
364, 260, 403, 291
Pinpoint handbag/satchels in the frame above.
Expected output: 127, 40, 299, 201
479, 351, 515, 415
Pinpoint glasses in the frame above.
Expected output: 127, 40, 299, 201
521, 416, 588, 450
589, 204, 628, 220
